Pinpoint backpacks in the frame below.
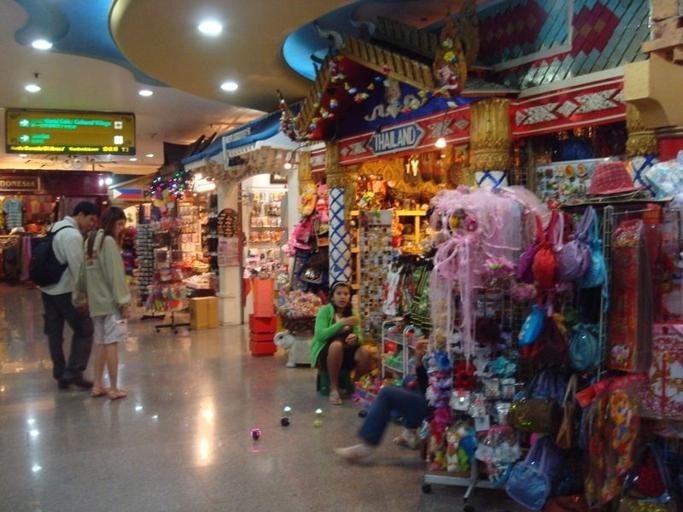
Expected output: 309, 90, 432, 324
29, 222, 75, 288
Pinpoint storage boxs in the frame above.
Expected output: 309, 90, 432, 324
188, 295, 218, 329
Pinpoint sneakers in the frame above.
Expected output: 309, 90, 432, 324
332, 443, 377, 466
392, 433, 420, 450
57, 367, 94, 391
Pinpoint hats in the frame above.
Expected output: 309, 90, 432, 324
585, 161, 641, 196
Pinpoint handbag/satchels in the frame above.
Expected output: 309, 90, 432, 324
504, 206, 609, 512
71, 240, 89, 305
617, 442, 676, 506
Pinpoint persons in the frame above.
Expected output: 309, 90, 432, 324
308, 279, 380, 406
330, 330, 450, 466
75, 205, 134, 401
29, 201, 99, 390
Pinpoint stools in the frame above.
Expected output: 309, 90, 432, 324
317, 367, 351, 397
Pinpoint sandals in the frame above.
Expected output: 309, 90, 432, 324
90, 387, 109, 397
108, 390, 128, 400
328, 389, 344, 405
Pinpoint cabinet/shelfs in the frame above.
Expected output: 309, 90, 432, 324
348, 209, 430, 290
380, 317, 426, 382
